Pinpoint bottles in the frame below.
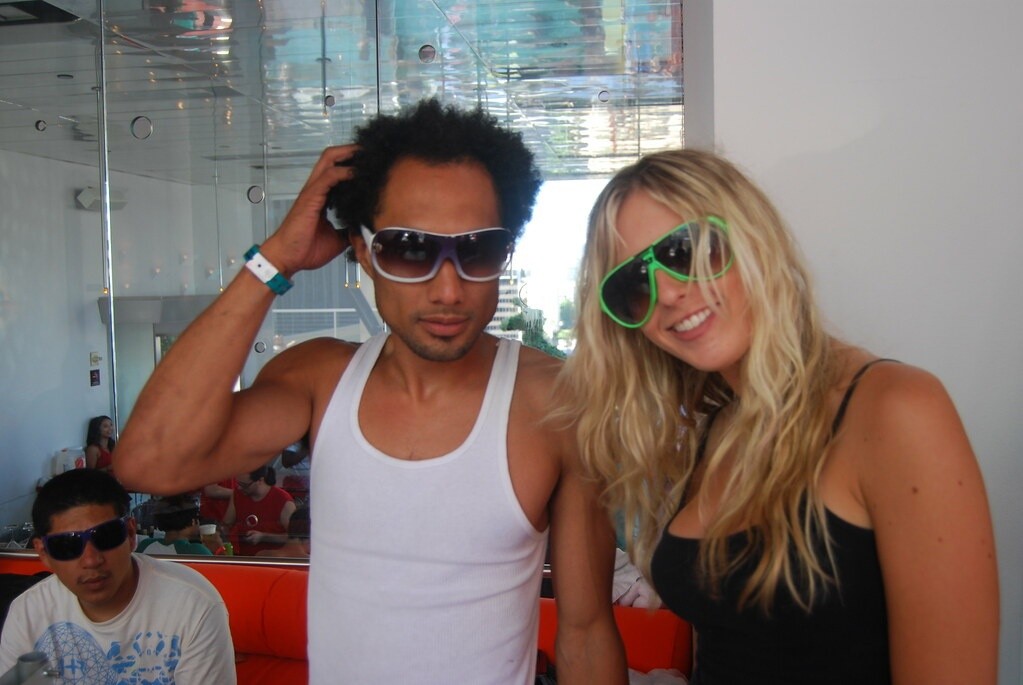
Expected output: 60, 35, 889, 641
135, 523, 155, 539
55, 445, 86, 475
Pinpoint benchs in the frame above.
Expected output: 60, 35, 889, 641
1, 548, 693, 685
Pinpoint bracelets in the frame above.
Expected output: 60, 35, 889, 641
243, 244, 292, 295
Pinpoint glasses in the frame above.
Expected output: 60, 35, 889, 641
234, 479, 255, 489
360, 227, 516, 282
599, 216, 734, 329
42, 514, 128, 561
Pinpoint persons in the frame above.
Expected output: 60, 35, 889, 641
0, 465, 238, 685
255, 504, 310, 558
84, 415, 115, 471
545, 150, 999, 685
198, 479, 239, 526
112, 97, 631, 685
135, 492, 214, 555
222, 465, 296, 555
